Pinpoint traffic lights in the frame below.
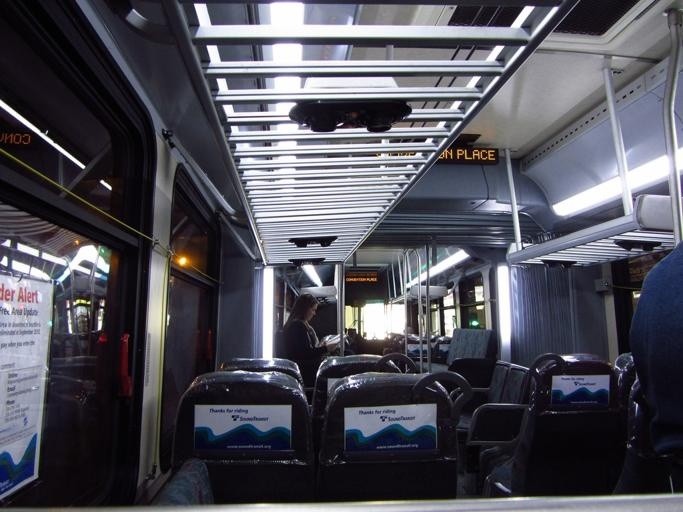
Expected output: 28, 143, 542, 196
467, 312, 479, 329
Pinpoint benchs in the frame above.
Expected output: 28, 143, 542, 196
414, 326, 534, 443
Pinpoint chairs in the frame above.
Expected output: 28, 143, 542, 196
481, 353, 683, 497
148, 352, 473, 503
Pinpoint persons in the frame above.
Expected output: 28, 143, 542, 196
283, 293, 338, 387
